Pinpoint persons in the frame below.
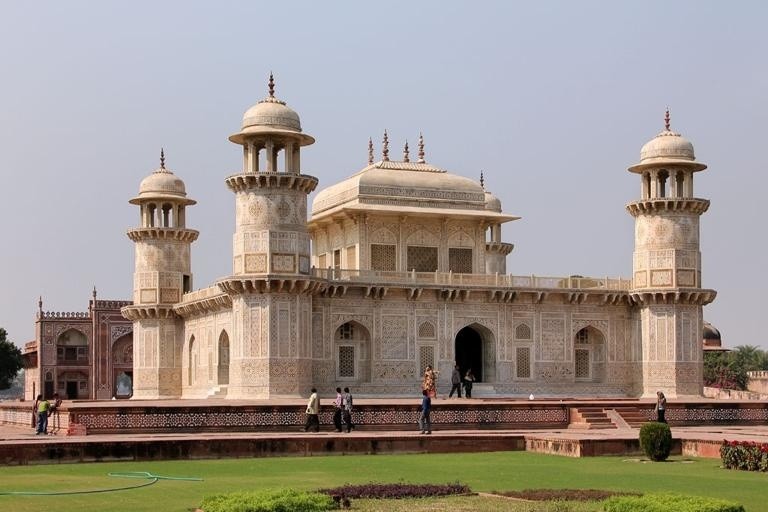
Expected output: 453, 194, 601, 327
447, 365, 463, 399
463, 367, 476, 398
653, 391, 666, 423
331, 387, 343, 433
417, 389, 431, 435
31, 394, 43, 432
46, 393, 62, 418
421, 366, 436, 400
33, 398, 51, 436
298, 388, 320, 433
341, 387, 355, 433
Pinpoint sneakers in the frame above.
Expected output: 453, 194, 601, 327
418, 429, 432, 435
300, 424, 356, 434
